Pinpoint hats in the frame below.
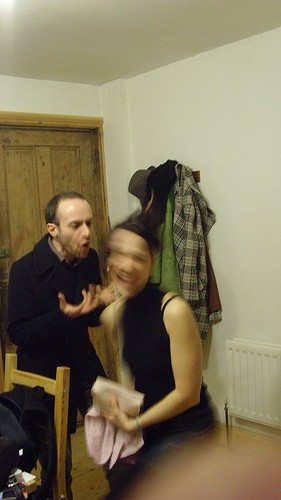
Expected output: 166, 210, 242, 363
129, 166, 155, 206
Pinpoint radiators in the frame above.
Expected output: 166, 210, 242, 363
225, 336, 280, 438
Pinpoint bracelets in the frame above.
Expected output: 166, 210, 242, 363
135, 416, 142, 433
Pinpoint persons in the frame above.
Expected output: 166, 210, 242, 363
92, 217, 225, 498
5, 191, 122, 500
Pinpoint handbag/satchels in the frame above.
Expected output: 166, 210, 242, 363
0, 385, 38, 492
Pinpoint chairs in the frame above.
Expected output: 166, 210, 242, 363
3, 353, 69, 498
